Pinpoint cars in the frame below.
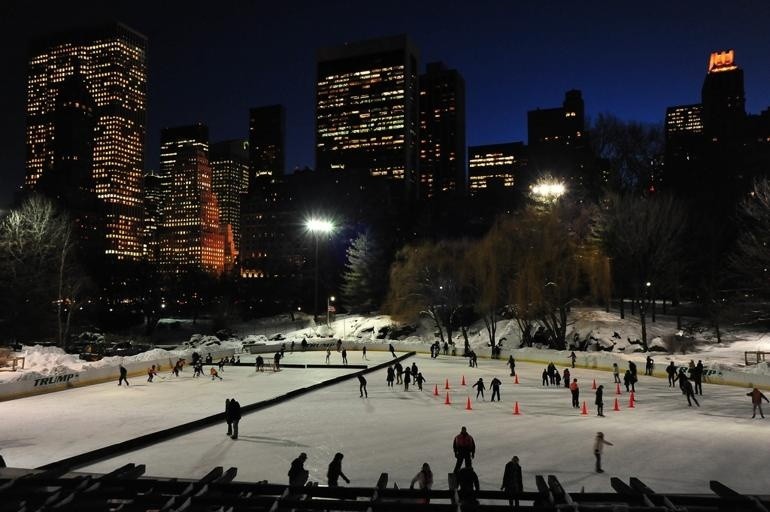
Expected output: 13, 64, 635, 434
107, 340, 135, 352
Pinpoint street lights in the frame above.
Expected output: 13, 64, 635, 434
305, 216, 341, 328
324, 295, 337, 331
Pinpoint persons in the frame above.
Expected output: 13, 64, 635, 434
747, 388, 769, 418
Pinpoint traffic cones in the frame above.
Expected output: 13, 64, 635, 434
590, 377, 638, 412
580, 400, 589, 417
429, 374, 474, 413
510, 372, 525, 387
512, 401, 522, 417
